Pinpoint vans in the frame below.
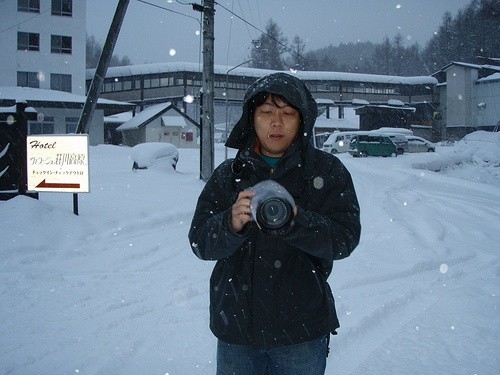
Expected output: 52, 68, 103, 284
348, 131, 398, 158
322, 131, 356, 154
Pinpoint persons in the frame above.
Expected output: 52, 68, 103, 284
187, 71, 363, 375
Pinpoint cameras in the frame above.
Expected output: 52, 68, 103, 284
247, 179, 297, 237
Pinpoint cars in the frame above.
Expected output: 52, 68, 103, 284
405, 135, 436, 153
385, 133, 409, 155
